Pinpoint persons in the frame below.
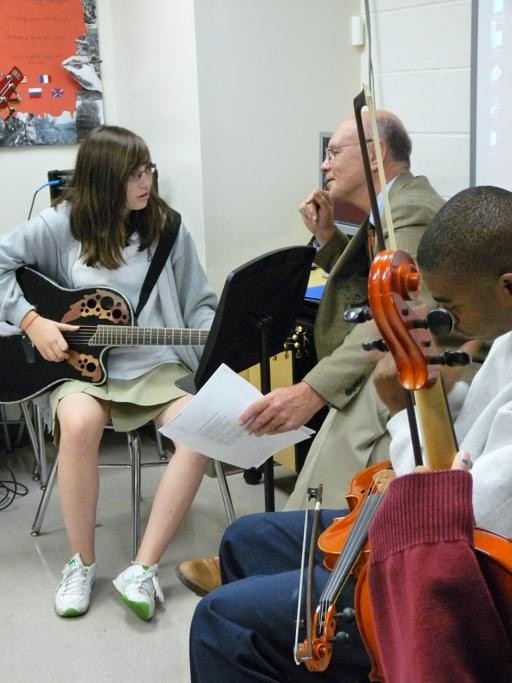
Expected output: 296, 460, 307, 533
177, 338, 474, 597
188, 186, 511, 681
238, 106, 452, 438
359, 464, 511, 683
1, 128, 222, 622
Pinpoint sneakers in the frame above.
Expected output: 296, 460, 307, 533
55, 553, 97, 617
112, 561, 164, 622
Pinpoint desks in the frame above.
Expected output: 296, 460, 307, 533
292, 283, 330, 474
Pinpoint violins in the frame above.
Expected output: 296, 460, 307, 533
299, 460, 400, 671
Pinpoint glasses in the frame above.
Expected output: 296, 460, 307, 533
326, 140, 373, 164
126, 164, 156, 181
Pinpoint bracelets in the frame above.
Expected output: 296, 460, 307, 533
22, 313, 40, 334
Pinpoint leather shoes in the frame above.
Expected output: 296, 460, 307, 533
176, 555, 221, 597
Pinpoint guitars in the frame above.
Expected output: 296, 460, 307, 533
0, 265, 209, 406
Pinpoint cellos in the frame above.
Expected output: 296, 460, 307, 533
354, 249, 511, 681
